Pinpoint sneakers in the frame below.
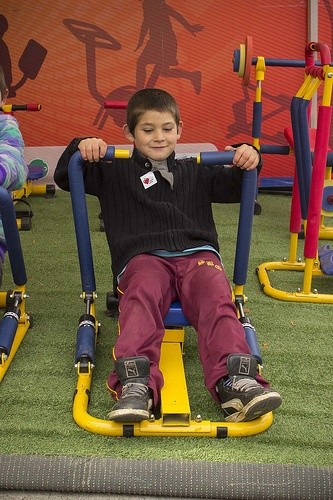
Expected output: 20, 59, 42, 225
216, 353, 282, 422
107, 356, 154, 421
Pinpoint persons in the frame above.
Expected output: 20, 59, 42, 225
55, 89, 283, 424
0, 66, 28, 293
